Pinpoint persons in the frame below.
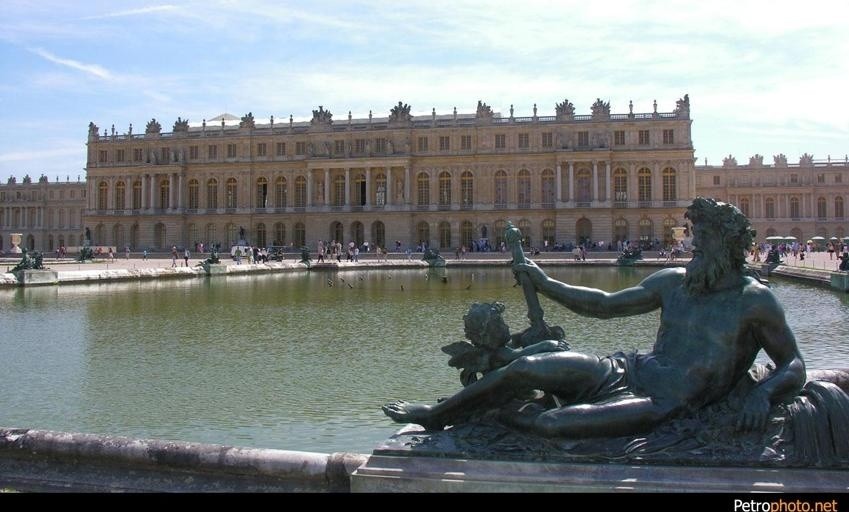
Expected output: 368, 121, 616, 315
9, 247, 31, 273
462, 300, 571, 374
380, 196, 808, 437
